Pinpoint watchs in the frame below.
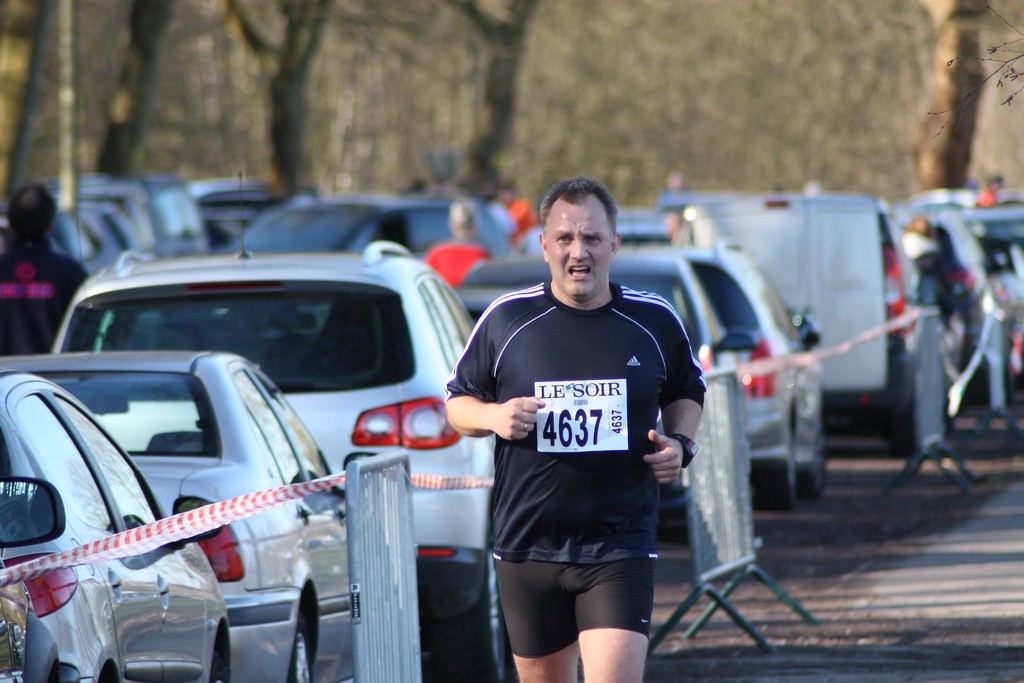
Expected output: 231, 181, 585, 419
669, 433, 700, 468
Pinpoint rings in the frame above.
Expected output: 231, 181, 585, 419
523, 422, 528, 432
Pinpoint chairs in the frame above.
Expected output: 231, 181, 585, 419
146, 430, 205, 457
313, 313, 374, 376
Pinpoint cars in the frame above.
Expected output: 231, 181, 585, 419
0, 473, 67, 683
1, 362, 234, 683
38, 185, 1023, 552
0, 346, 351, 683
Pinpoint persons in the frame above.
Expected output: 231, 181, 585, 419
0, 179, 88, 357
972, 174, 1004, 208
446, 175, 706, 683
424, 177, 546, 289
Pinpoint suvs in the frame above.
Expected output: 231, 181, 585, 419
48, 234, 518, 683
